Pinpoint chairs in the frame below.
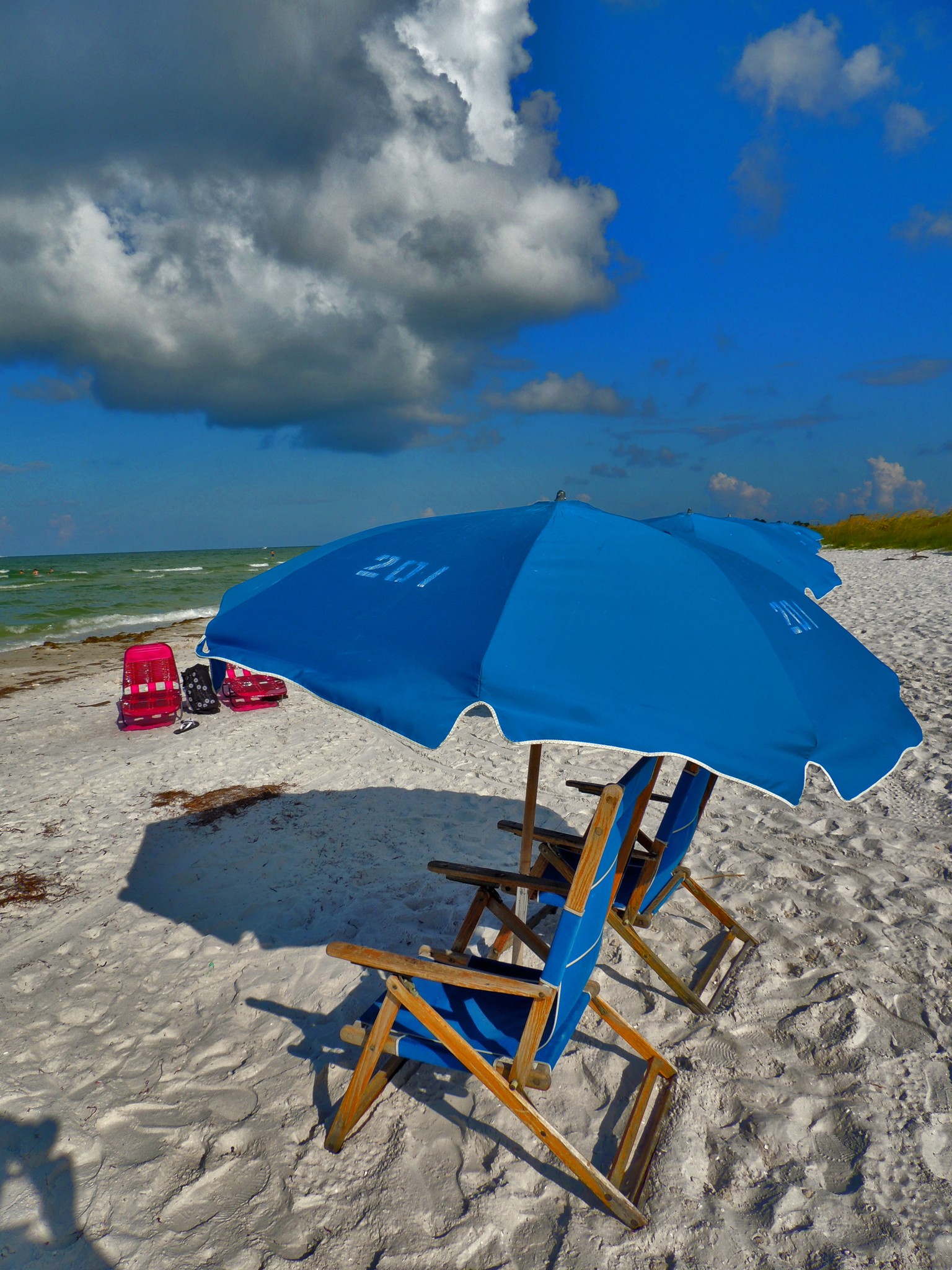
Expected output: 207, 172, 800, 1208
222, 662, 287, 712
324, 755, 761, 1230
119, 642, 183, 732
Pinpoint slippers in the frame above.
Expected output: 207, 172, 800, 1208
173, 722, 200, 734
180, 717, 199, 725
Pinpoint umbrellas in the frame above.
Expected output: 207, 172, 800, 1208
636, 509, 843, 601
196, 497, 924, 966
